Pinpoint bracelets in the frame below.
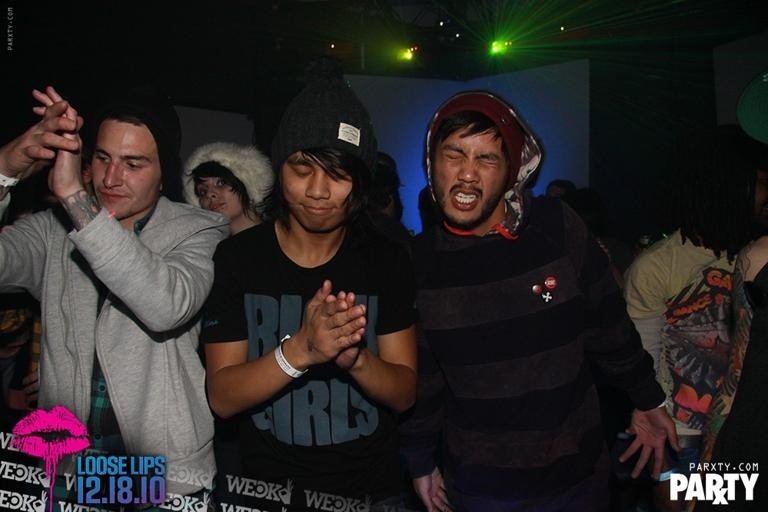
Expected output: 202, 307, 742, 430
0, 173, 19, 188
273, 332, 309, 383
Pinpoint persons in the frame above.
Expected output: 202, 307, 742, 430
541, 178, 579, 201
0, 141, 96, 413
0, 80, 221, 511
175, 139, 283, 237
614, 119, 765, 512
408, 90, 683, 512
560, 183, 629, 289
202, 74, 422, 511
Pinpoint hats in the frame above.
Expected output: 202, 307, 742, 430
90, 90, 181, 189
271, 75, 379, 172
425, 90, 525, 194
176, 137, 275, 216
